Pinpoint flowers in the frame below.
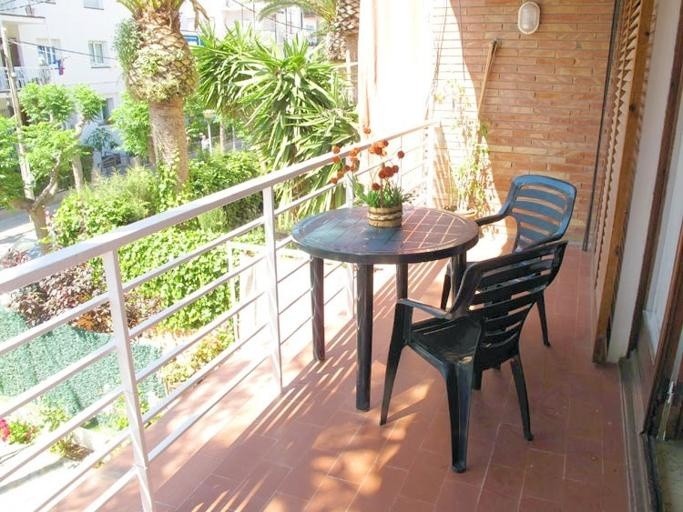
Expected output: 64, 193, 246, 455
329, 127, 420, 207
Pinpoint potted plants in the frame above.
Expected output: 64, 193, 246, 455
433, 78, 491, 220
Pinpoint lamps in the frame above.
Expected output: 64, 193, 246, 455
518, 1, 540, 34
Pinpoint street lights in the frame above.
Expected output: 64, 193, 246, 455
203, 107, 214, 156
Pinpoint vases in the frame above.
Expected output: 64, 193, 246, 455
367, 203, 404, 227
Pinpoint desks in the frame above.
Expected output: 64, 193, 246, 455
288, 205, 476, 411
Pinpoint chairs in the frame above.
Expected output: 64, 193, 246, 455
379, 241, 568, 474
442, 174, 576, 370
